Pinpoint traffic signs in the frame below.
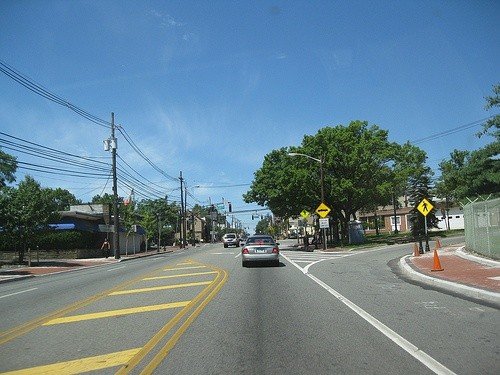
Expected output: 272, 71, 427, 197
215, 202, 225, 210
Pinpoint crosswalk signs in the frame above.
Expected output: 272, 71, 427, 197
416, 198, 434, 216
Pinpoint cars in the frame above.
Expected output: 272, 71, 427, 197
240, 234, 280, 266
177, 237, 189, 246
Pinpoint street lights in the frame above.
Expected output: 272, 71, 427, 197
288, 152, 326, 251
185, 185, 200, 246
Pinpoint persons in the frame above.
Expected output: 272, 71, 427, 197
101, 238, 110, 258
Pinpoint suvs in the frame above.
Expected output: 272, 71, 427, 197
223, 233, 240, 248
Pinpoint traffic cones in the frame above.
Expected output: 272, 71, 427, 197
413, 242, 420, 256
436, 239, 441, 248
431, 249, 444, 271
